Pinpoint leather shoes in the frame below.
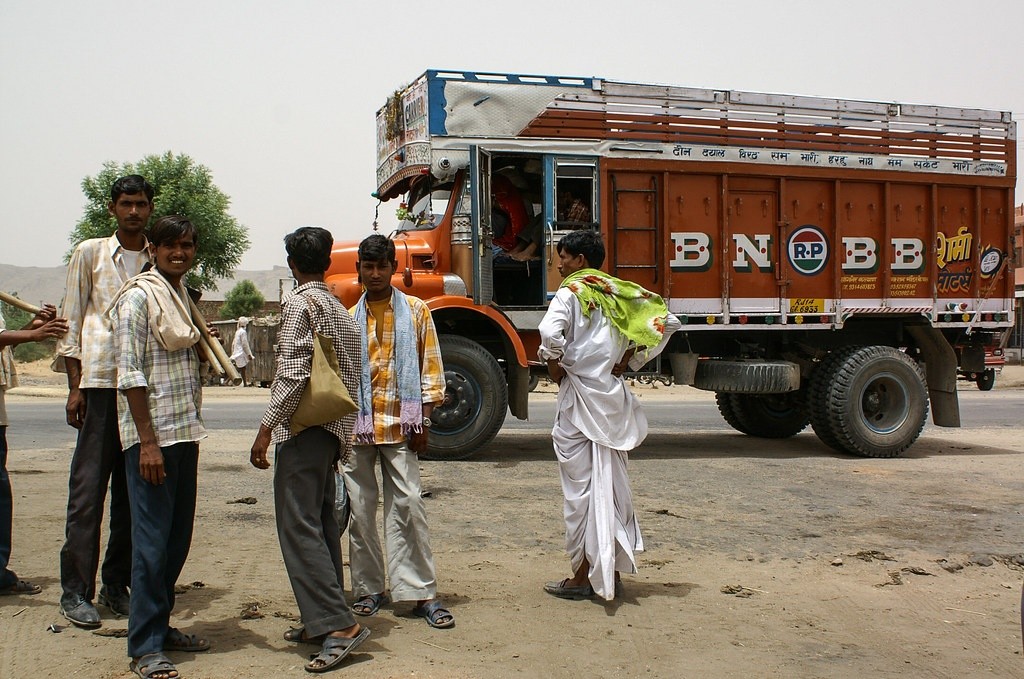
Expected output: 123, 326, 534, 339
615, 580, 624, 597
98, 583, 131, 617
543, 578, 595, 599
60, 593, 102, 626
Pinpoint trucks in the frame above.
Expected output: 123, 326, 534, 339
322, 69, 1020, 459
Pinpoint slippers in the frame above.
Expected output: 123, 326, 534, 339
129, 651, 181, 679
351, 591, 388, 616
163, 626, 211, 652
284, 625, 311, 643
304, 627, 371, 673
413, 598, 455, 628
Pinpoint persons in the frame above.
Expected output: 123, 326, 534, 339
51, 174, 156, 624
0, 302, 69, 595
537, 230, 681, 599
101, 215, 225, 679
504, 181, 592, 262
250, 226, 372, 673
347, 233, 456, 629
224, 316, 255, 386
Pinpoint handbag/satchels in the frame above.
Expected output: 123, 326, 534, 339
289, 294, 361, 436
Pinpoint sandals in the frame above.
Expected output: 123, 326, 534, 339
0, 579, 41, 595
